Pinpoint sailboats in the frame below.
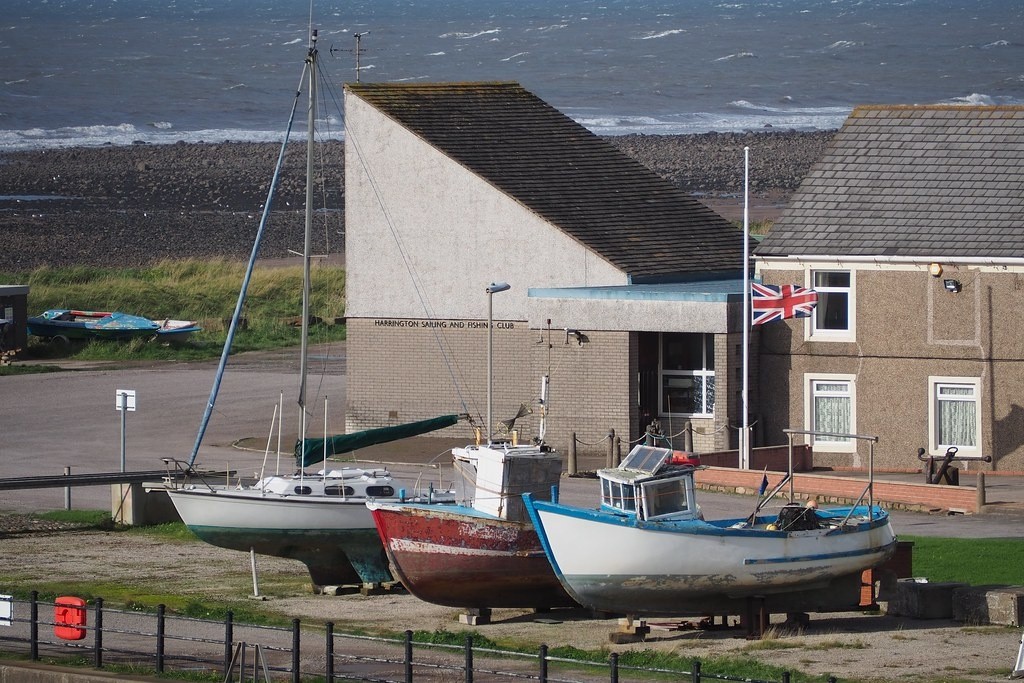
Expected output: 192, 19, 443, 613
137, 1, 499, 594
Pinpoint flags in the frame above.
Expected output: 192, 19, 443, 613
750, 282, 820, 326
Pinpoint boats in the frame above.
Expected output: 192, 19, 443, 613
360, 441, 705, 611
152, 319, 203, 345
26, 307, 161, 341
517, 421, 900, 619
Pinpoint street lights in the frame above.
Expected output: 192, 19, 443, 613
486, 282, 511, 445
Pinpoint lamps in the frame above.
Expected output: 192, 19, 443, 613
944, 279, 962, 294
569, 330, 585, 346
